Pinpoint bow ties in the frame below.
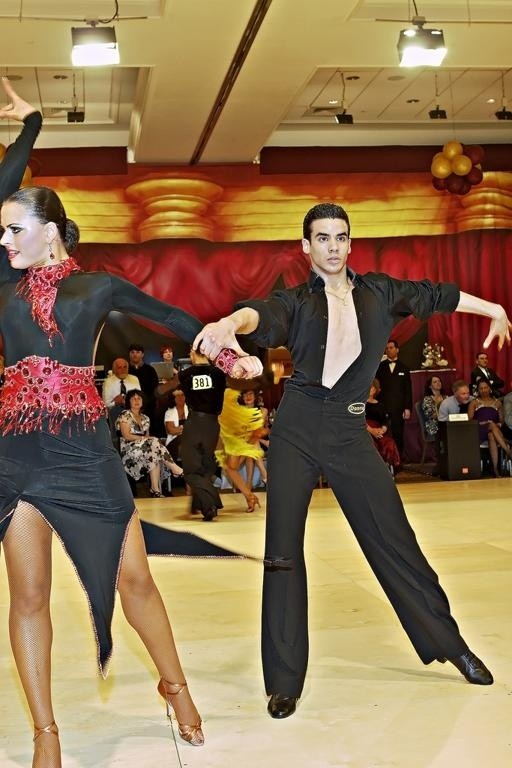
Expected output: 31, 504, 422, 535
388, 360, 397, 363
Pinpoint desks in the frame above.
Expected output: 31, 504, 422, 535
432, 416, 481, 481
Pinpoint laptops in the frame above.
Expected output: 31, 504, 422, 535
151, 361, 174, 379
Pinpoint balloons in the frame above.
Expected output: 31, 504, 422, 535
429, 139, 486, 197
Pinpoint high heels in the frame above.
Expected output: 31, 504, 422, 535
32, 719, 58, 741
157, 677, 204, 745
246, 495, 261, 512
149, 487, 165, 497
173, 472, 184, 480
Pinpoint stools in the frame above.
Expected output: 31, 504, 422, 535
151, 453, 326, 493
477, 436, 512, 476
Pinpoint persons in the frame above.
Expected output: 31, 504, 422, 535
192, 202, 512, 719
363, 337, 512, 481
0, 74, 271, 768
102, 344, 271, 522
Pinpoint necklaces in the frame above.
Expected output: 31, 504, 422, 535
325, 277, 353, 308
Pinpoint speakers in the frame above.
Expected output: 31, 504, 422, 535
438, 420, 481, 480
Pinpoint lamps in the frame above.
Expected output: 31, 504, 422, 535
62, 1, 128, 71
393, 1, 449, 68
428, 72, 448, 118
495, 71, 512, 121
61, 70, 86, 124
334, 70, 355, 124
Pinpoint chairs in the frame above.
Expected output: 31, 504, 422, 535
415, 402, 439, 464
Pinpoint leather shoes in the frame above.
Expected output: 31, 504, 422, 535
448, 649, 494, 684
268, 693, 296, 718
202, 507, 217, 520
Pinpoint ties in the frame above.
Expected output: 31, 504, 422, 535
485, 368, 490, 379
119, 379, 126, 394
